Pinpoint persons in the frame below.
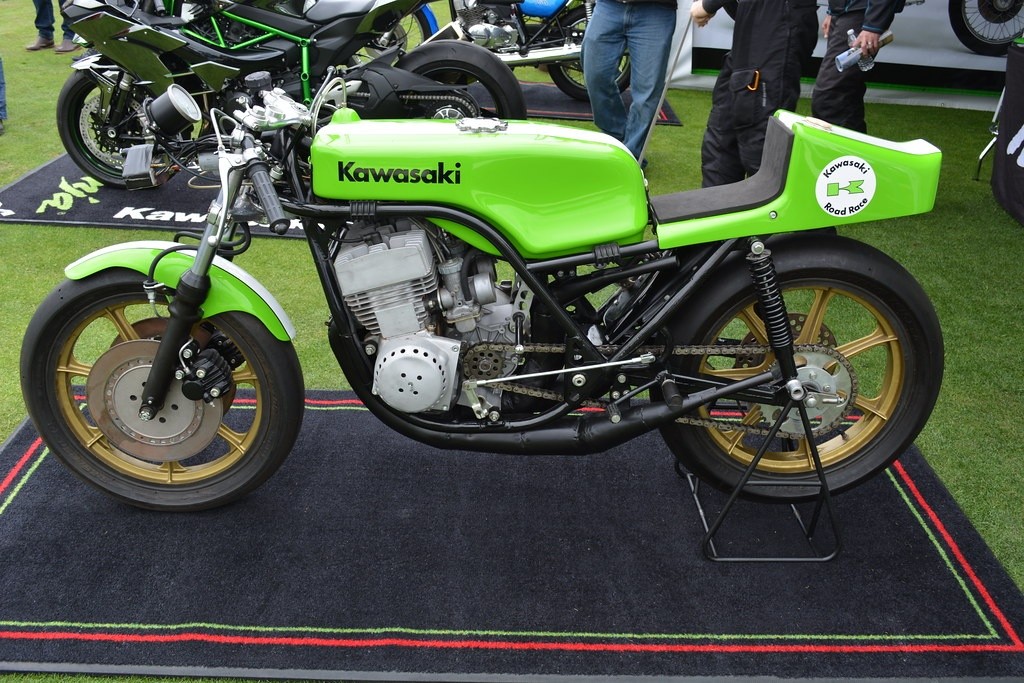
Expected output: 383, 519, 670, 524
810, 0, 905, 134
579, 0, 678, 167
0, 58, 7, 132
692, 0, 819, 188
71, 46, 98, 60
25, 0, 80, 51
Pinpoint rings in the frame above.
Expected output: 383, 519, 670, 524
868, 42, 872, 43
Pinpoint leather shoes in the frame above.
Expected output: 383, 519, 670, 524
26, 36, 55, 50
54, 39, 82, 53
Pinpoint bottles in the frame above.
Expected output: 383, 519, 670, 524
847, 28, 875, 71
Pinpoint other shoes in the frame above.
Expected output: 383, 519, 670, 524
72, 48, 101, 61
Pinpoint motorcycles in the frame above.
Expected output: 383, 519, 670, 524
18, 65, 949, 517
55, 0, 531, 189
349, 0, 628, 104
